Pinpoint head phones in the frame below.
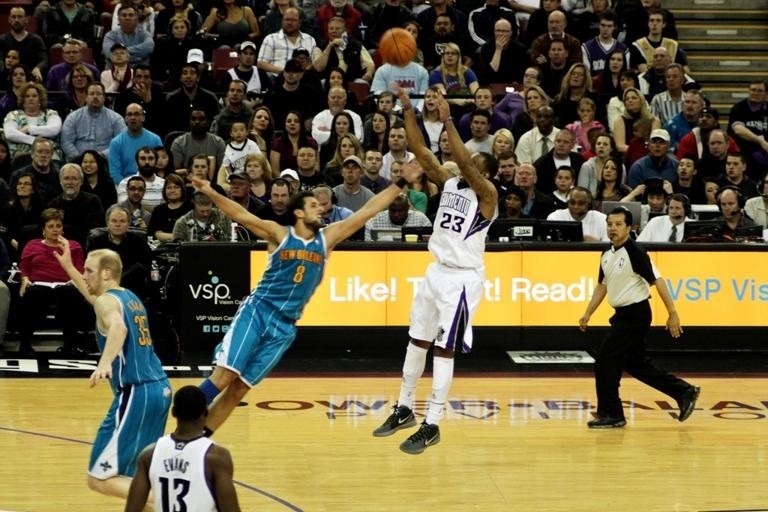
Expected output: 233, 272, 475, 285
307, 184, 338, 205
565, 187, 594, 210
713, 186, 745, 209
190, 191, 216, 209
757, 179, 764, 196
265, 177, 294, 197
665, 194, 691, 215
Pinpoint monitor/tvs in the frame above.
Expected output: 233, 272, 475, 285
488, 218, 537, 242
370, 229, 404, 241
681, 221, 728, 243
537, 220, 584, 243
401, 226, 433, 242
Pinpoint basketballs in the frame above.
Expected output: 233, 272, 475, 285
378, 28, 415, 68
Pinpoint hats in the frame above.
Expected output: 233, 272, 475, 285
241, 41, 256, 51
110, 42, 127, 52
228, 171, 251, 181
285, 60, 302, 72
187, 48, 203, 64
650, 129, 671, 142
343, 154, 363, 170
280, 168, 299, 181
292, 47, 310, 58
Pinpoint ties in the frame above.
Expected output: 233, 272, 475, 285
541, 137, 548, 156
669, 226, 678, 242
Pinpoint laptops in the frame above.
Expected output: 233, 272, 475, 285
602, 202, 641, 228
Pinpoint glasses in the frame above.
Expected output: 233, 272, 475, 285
18, 182, 32, 185
494, 29, 509, 33
126, 112, 143, 117
445, 51, 458, 55
128, 187, 146, 192
570, 71, 586, 77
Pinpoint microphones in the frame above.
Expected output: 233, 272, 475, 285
609, 236, 614, 243
674, 216, 682, 219
578, 211, 589, 217
730, 209, 742, 217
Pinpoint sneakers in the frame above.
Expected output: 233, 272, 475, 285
373, 405, 417, 437
587, 416, 627, 429
399, 419, 441, 454
679, 386, 700, 422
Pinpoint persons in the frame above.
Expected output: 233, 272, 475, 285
387, 161, 428, 214
366, 0, 693, 96
123, 386, 240, 512
150, 175, 195, 242
458, 86, 513, 142
498, 85, 768, 241
373, 83, 500, 454
87, 205, 154, 304
415, 85, 448, 160
366, 110, 392, 155
186, 159, 425, 440
279, 168, 304, 197
433, 130, 456, 165
174, 2, 364, 164
579, 207, 700, 429
312, 187, 353, 226
173, 190, 232, 243
364, 91, 406, 144
360, 147, 391, 192
363, 193, 430, 241
379, 122, 416, 182
324, 134, 365, 186
245, 155, 275, 205
333, 156, 376, 212
182, 154, 226, 210
226, 171, 266, 221
0, 240, 13, 351
295, 148, 328, 192
461, 111, 497, 157
491, 128, 515, 160
253, 180, 302, 226
15, 207, 84, 356
1, 1, 173, 247
497, 152, 522, 194
51, 233, 171, 510
